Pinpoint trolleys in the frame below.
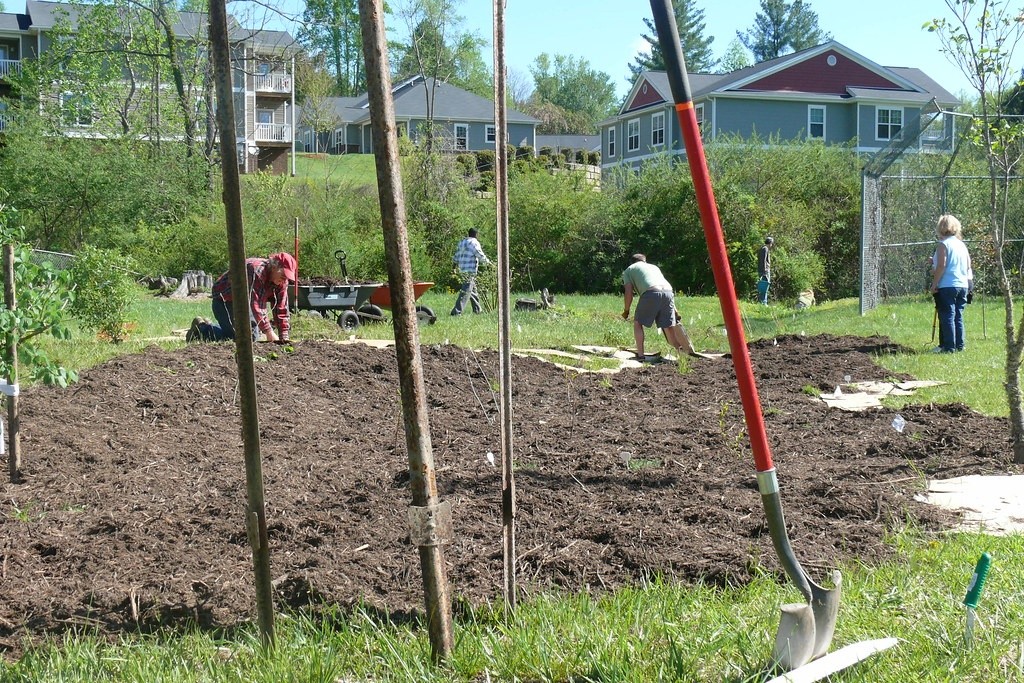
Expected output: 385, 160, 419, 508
287, 281, 386, 332
365, 279, 437, 325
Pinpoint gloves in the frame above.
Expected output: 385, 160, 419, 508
621, 309, 630, 321
675, 311, 681, 322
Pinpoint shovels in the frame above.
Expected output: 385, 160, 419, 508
651, 1, 843, 674
923, 303, 938, 350
289, 217, 303, 318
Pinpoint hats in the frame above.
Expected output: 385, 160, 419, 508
271, 251, 297, 281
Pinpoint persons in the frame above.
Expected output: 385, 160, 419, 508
621, 253, 695, 361
450, 226, 495, 316
927, 212, 974, 354
757, 236, 776, 307
795, 287, 816, 308
186, 252, 297, 344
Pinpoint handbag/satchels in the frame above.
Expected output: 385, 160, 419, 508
967, 279, 973, 304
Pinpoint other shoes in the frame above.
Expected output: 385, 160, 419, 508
628, 355, 645, 362
186, 316, 212, 344
